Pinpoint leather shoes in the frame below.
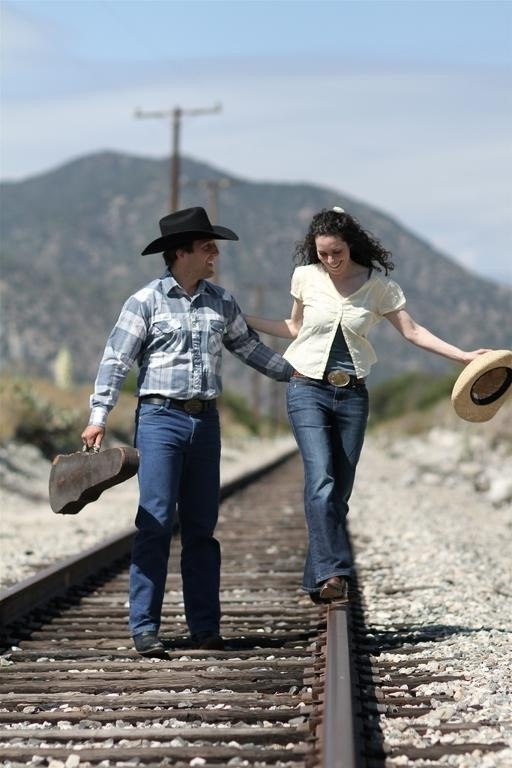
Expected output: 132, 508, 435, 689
134, 632, 166, 656
320, 577, 350, 602
194, 630, 230, 652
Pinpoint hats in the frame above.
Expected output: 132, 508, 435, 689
139, 206, 241, 253
449, 348, 512, 423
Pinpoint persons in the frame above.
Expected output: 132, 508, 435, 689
243, 207, 494, 605
81, 207, 296, 657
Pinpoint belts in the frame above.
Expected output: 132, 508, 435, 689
292, 372, 366, 388
140, 394, 217, 415
